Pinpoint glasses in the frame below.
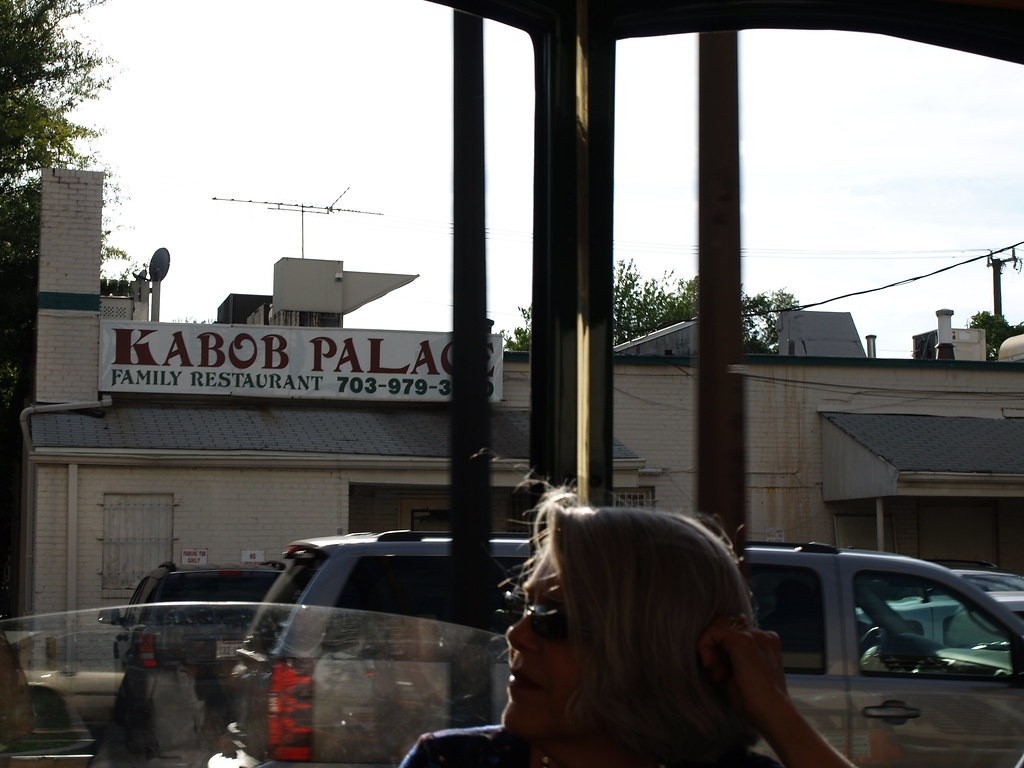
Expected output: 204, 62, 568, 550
502, 590, 596, 644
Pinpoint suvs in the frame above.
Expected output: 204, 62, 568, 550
13, 527, 1024, 768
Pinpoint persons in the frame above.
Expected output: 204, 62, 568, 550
395, 502, 856, 768
